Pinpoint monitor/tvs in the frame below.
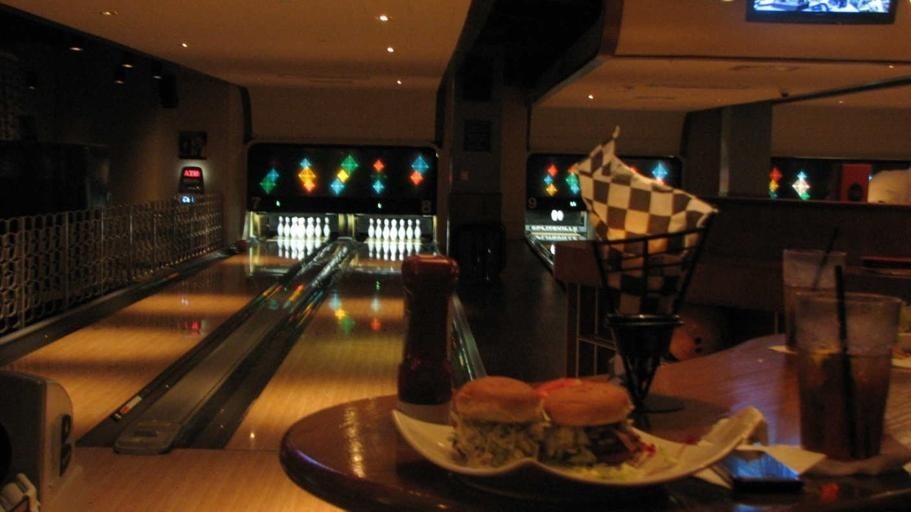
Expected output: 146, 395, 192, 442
746, 0, 896, 24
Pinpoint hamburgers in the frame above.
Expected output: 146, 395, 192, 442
447, 376, 542, 467
536, 376, 642, 473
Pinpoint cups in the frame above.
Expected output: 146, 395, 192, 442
792, 288, 902, 462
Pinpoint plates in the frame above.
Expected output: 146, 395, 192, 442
384, 407, 763, 485
